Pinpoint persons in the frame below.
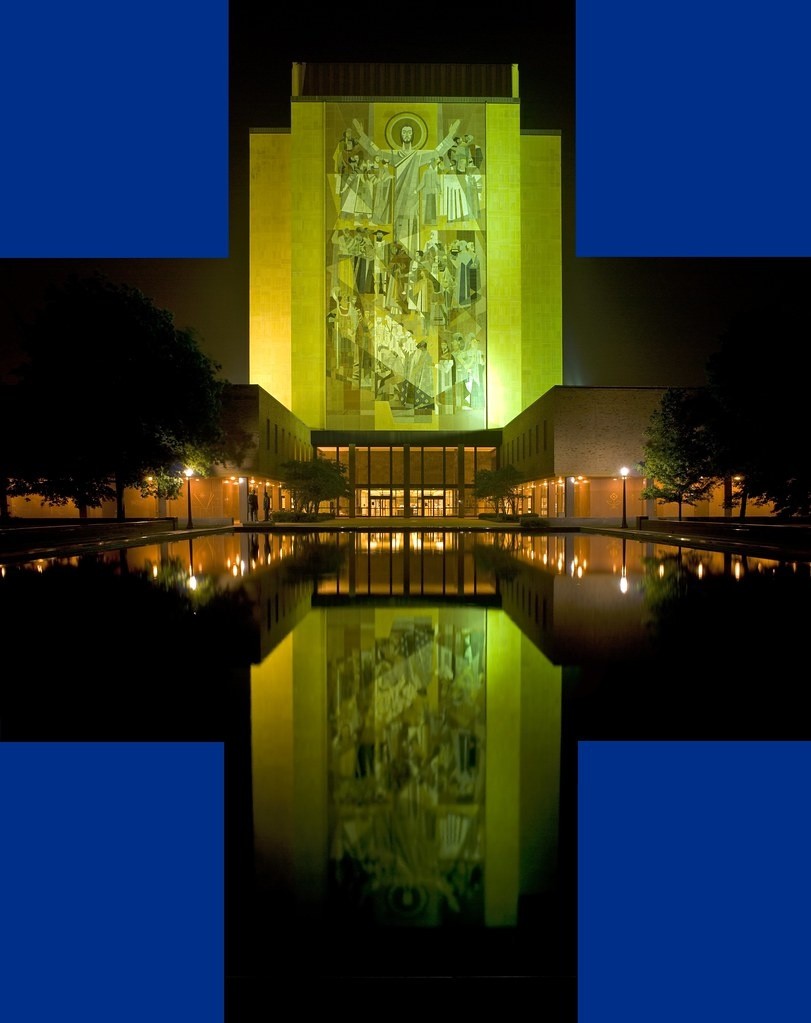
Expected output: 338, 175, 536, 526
251, 534, 259, 562
263, 492, 270, 521
263, 534, 271, 564
248, 490, 259, 521
